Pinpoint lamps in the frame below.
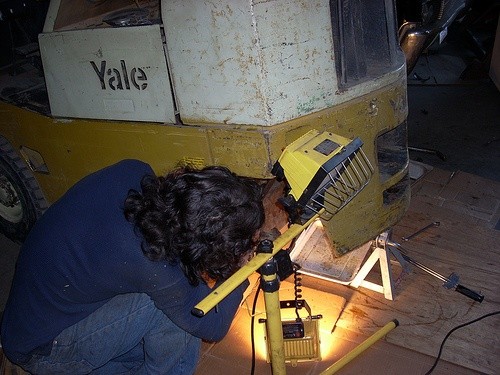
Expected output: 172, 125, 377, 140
190, 128, 375, 375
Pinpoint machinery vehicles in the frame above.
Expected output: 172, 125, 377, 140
0, 0, 413, 258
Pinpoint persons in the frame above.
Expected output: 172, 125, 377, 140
0, 156, 266, 375
